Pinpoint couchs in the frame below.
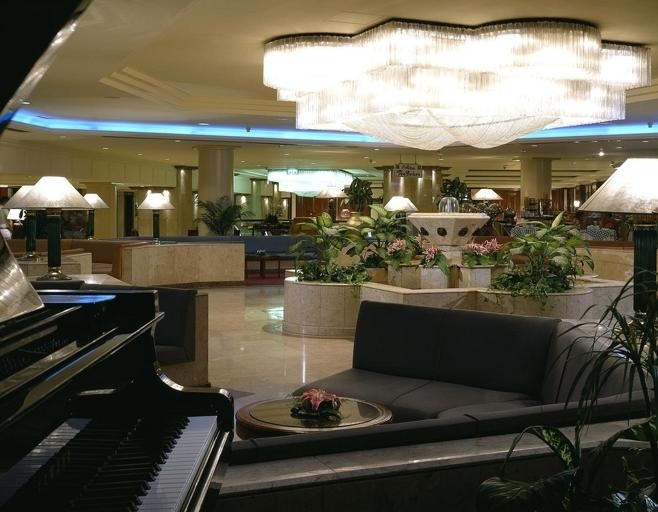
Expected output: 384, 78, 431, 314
290, 299, 561, 423
214, 391, 656, 510
29, 282, 208, 388
6, 239, 148, 280
435, 320, 656, 421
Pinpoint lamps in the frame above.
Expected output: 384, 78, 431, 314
137, 193, 176, 245
401, 198, 419, 232
384, 195, 413, 217
263, 16, 652, 151
471, 187, 505, 207
577, 157, 658, 341
9, 176, 94, 280
83, 193, 110, 239
1, 186, 46, 259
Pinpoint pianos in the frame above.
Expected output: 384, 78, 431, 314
0, 287, 236, 512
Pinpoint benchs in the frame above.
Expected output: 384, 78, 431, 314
156, 236, 319, 277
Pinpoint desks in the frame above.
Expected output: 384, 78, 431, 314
108, 242, 245, 288
18, 252, 92, 278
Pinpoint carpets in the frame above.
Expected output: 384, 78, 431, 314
243, 268, 295, 285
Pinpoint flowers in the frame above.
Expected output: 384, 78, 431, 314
385, 237, 505, 276
